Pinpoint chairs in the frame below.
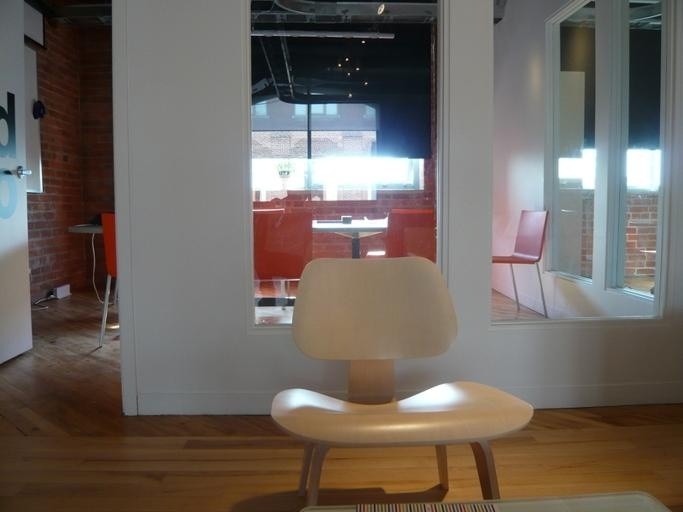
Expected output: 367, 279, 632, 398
271, 256, 534, 507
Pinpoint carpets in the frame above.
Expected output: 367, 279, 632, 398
299, 491, 673, 512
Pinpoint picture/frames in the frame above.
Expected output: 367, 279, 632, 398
24, 0, 46, 48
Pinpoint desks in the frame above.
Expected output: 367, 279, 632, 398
68, 224, 103, 234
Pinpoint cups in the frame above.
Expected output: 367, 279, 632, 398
340, 216, 352, 225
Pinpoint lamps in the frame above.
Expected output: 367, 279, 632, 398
377, 4, 392, 16
15, 165, 32, 178
